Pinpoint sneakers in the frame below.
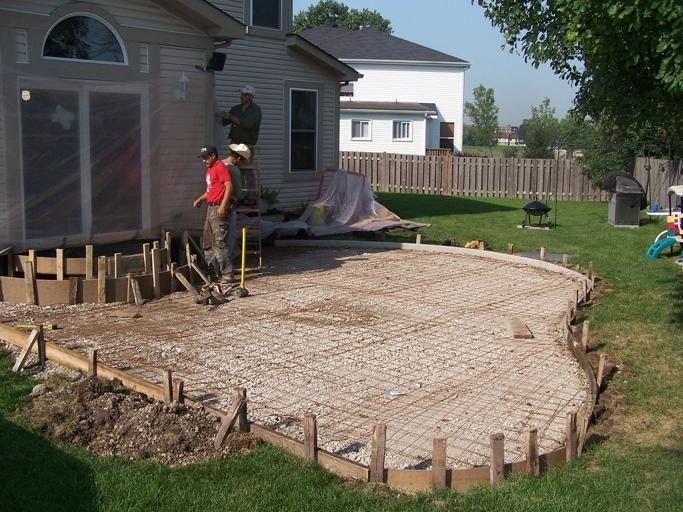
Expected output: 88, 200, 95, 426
221, 275, 234, 284
240, 199, 258, 209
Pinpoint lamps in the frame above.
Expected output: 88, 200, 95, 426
175, 69, 189, 102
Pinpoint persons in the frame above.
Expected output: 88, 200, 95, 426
219, 144, 251, 253
219, 86, 261, 205
192, 144, 234, 285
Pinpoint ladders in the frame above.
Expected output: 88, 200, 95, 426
230, 144, 262, 277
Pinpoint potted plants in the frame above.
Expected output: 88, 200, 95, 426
257, 182, 280, 214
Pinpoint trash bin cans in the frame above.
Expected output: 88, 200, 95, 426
608, 175, 642, 228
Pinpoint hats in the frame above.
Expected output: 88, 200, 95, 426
196, 145, 216, 157
239, 85, 255, 96
228, 143, 250, 161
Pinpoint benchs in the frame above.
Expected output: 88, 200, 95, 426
646, 211, 682, 221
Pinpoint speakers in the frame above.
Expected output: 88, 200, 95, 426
209, 52, 226, 71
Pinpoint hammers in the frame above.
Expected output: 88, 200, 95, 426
236, 228, 249, 298
16, 324, 57, 330
